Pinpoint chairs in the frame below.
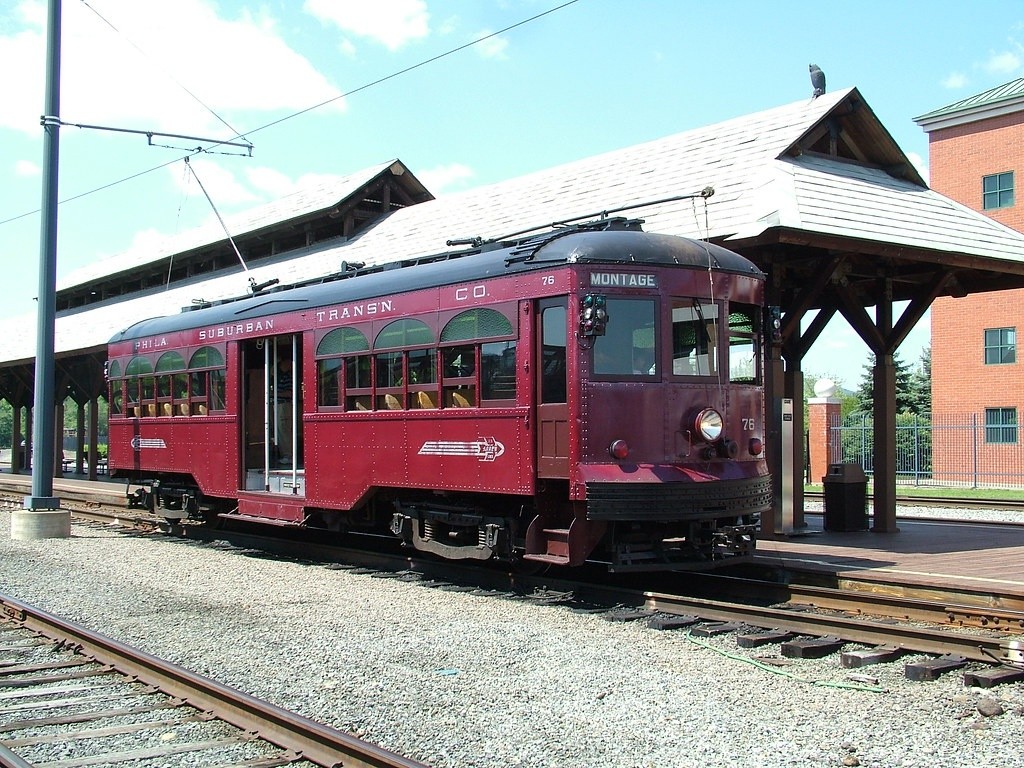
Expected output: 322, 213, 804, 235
354, 389, 475, 409
130, 405, 207, 418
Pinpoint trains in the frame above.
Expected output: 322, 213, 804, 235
104, 216, 776, 571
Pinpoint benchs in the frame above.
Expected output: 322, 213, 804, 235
96, 461, 108, 474
62, 459, 74, 474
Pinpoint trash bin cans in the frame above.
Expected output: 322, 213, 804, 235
822, 463, 869, 532
